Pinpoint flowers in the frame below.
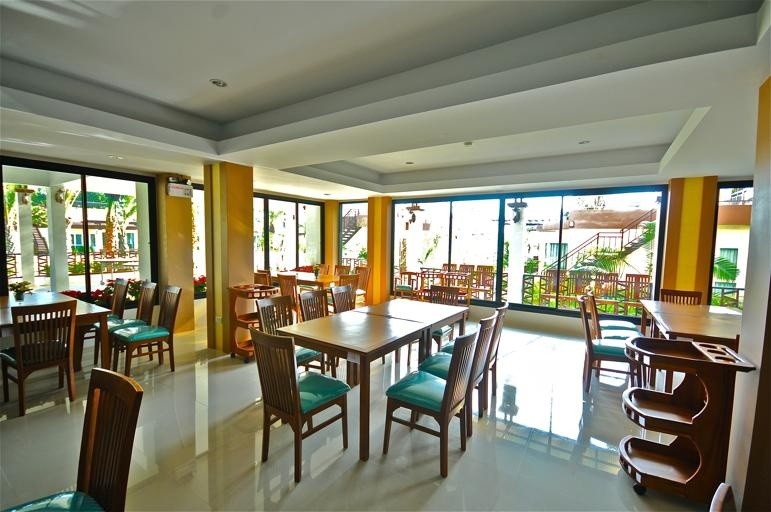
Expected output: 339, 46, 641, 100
8, 280, 34, 294
59, 277, 145, 310
193, 273, 208, 293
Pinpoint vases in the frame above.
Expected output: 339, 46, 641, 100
193, 291, 206, 300
14, 292, 25, 302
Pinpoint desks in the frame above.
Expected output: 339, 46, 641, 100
1, 289, 112, 381
636, 297, 744, 395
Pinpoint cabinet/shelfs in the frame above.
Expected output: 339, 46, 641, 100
227, 283, 282, 363
617, 331, 757, 506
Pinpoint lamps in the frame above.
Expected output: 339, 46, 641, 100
166, 174, 193, 199
54, 188, 65, 204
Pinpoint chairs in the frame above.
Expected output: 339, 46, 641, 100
419, 302, 509, 437
538, 269, 620, 316
657, 287, 705, 374
254, 264, 371, 309
623, 274, 650, 315
586, 288, 645, 339
250, 327, 351, 483
0, 298, 78, 418
382, 332, 479, 478
299, 290, 328, 321
255, 294, 326, 375
577, 295, 635, 394
75, 276, 184, 377
330, 285, 355, 313
394, 264, 494, 306
0, 365, 146, 511
